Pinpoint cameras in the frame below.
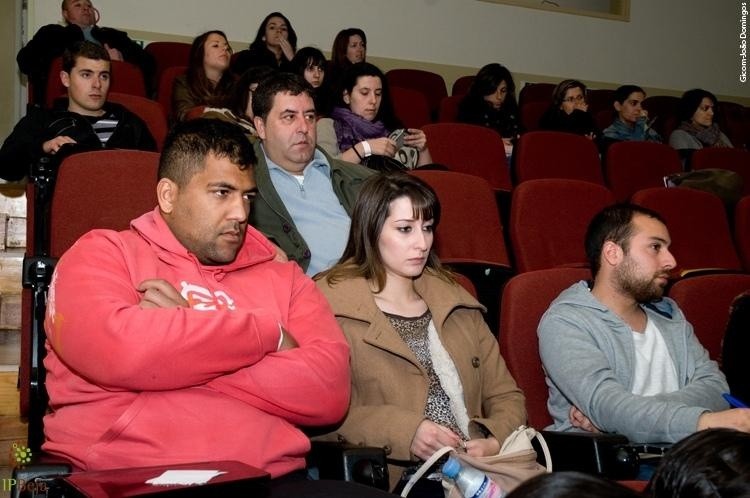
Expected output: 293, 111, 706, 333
389, 127, 410, 152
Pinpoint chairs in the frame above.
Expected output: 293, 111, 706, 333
11, 42, 750, 498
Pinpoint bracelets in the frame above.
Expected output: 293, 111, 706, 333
361, 140, 371, 157
351, 146, 363, 160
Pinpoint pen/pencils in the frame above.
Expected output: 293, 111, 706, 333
722, 393, 748, 408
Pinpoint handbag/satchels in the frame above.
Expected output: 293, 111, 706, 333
664, 168, 737, 197
386, 128, 418, 170
442, 425, 546, 498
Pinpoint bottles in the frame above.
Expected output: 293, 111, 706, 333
441, 455, 506, 498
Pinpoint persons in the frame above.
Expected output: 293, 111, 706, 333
0, 40, 158, 183
308, 170, 549, 498
668, 88, 734, 172
315, 59, 434, 167
505, 472, 639, 498
642, 427, 750, 498
18, 0, 157, 108
537, 202, 750, 482
173, 11, 367, 123
455, 63, 524, 168
247, 72, 377, 278
603, 85, 662, 144
37, 118, 404, 498
538, 79, 603, 169
